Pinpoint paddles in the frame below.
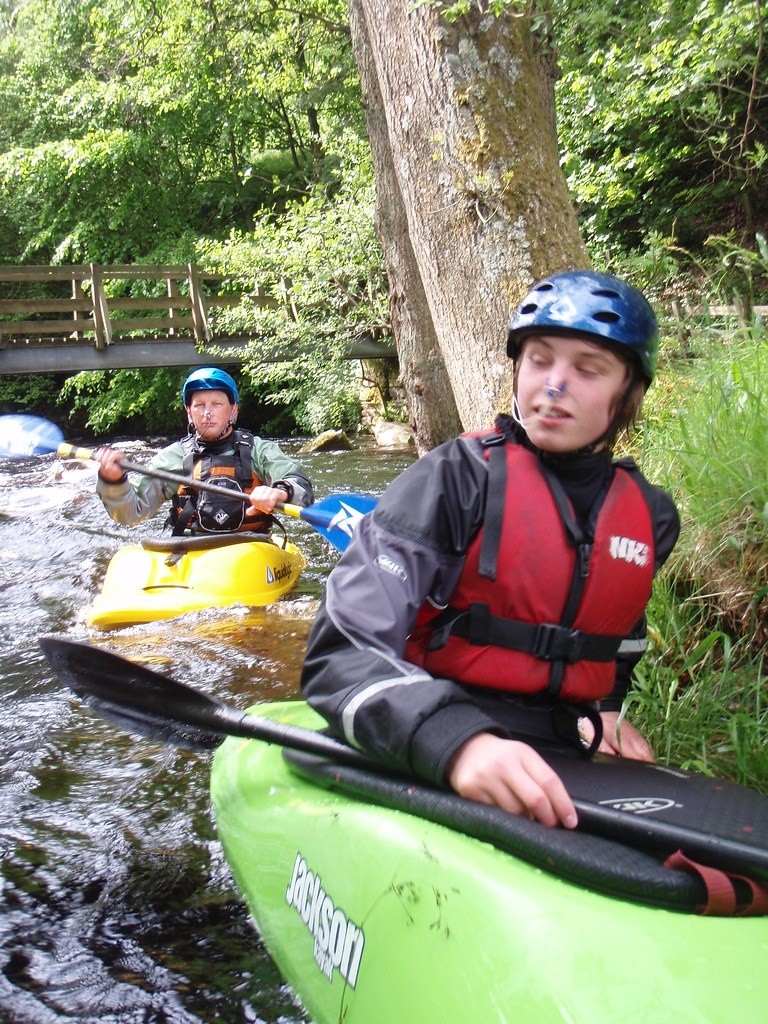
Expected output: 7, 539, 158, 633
37, 632, 767, 880
0, 413, 378, 554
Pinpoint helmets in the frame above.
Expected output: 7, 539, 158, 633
183, 368, 239, 405
506, 271, 659, 396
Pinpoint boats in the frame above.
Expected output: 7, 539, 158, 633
209, 697, 768, 1024
89, 531, 304, 634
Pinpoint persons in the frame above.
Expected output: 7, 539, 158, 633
295, 268, 679, 828
95, 366, 314, 538
